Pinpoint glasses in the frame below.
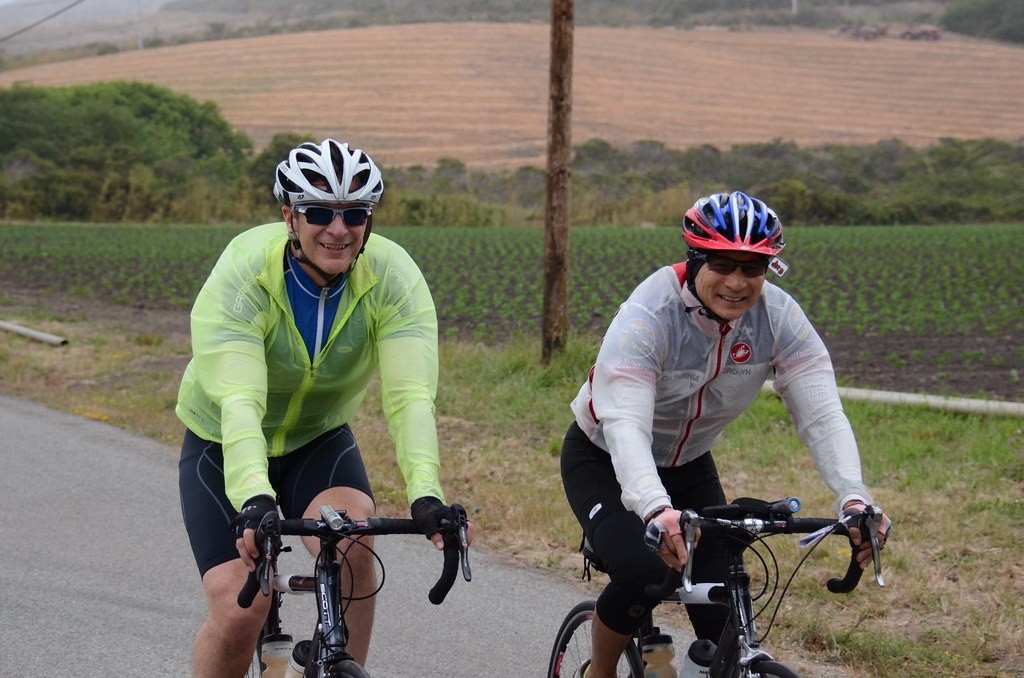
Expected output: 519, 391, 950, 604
692, 250, 769, 278
293, 204, 373, 227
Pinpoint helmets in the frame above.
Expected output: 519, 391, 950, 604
682, 191, 787, 257
273, 138, 385, 207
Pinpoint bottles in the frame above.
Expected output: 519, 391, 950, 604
679, 639, 717, 678
261, 626, 294, 678
642, 626, 676, 678
285, 640, 314, 678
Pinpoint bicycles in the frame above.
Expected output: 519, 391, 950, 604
545, 496, 887, 678
221, 499, 473, 678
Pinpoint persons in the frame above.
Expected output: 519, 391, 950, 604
173, 138, 473, 677
559, 190, 893, 677
